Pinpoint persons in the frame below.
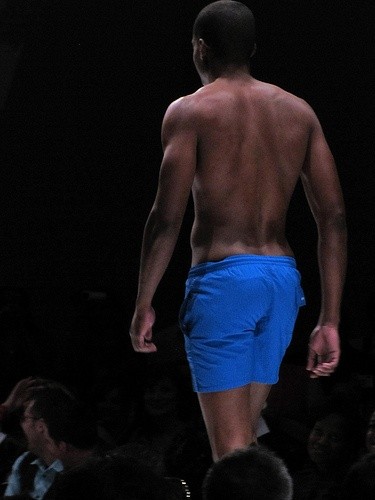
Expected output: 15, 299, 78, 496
130, 0, 348, 463
0, 378, 375, 500
